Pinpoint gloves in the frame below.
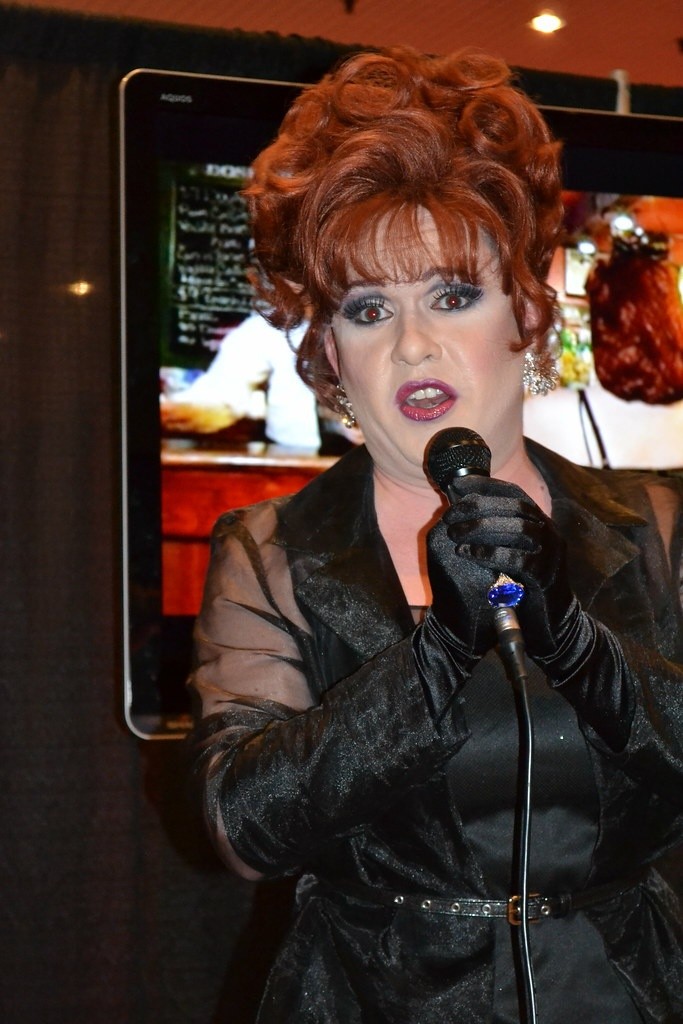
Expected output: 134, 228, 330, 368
445, 474, 635, 753
410, 518, 496, 727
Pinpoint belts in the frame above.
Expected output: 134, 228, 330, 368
327, 877, 639, 926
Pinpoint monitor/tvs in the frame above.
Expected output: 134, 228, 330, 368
113, 68, 683, 740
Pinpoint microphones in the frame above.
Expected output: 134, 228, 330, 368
428, 427, 528, 681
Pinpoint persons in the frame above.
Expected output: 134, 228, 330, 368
169, 267, 322, 460
520, 255, 683, 469
191, 47, 683, 1024
